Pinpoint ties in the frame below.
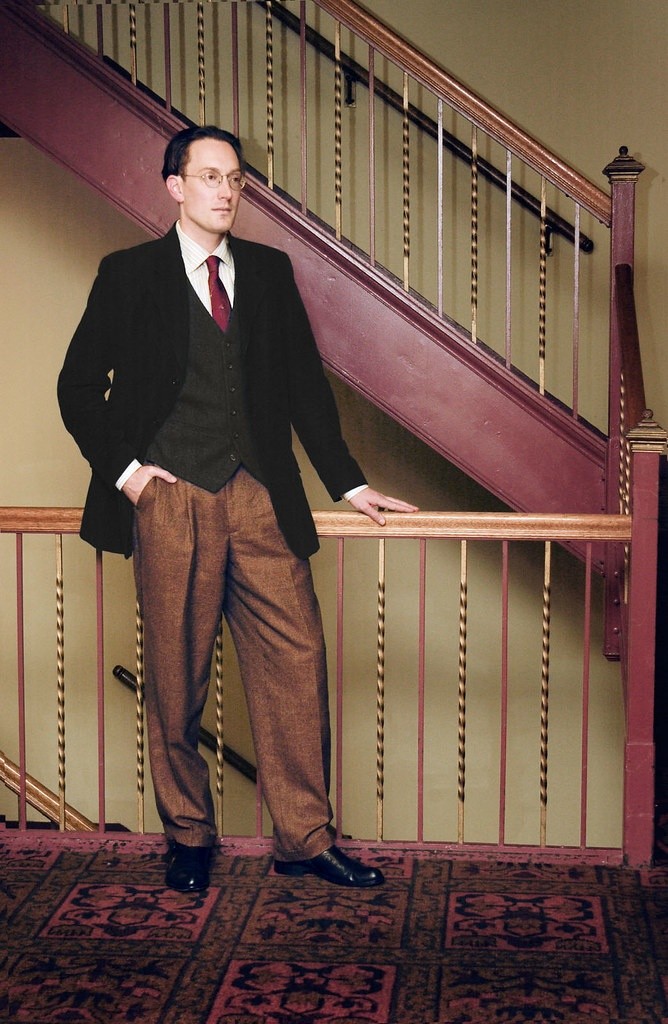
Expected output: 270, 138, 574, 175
205, 255, 231, 333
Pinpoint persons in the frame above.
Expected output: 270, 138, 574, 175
56, 127, 417, 891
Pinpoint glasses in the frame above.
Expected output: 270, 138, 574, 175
184, 171, 247, 190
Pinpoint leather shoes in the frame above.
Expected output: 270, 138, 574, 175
273, 845, 385, 887
166, 843, 213, 892
